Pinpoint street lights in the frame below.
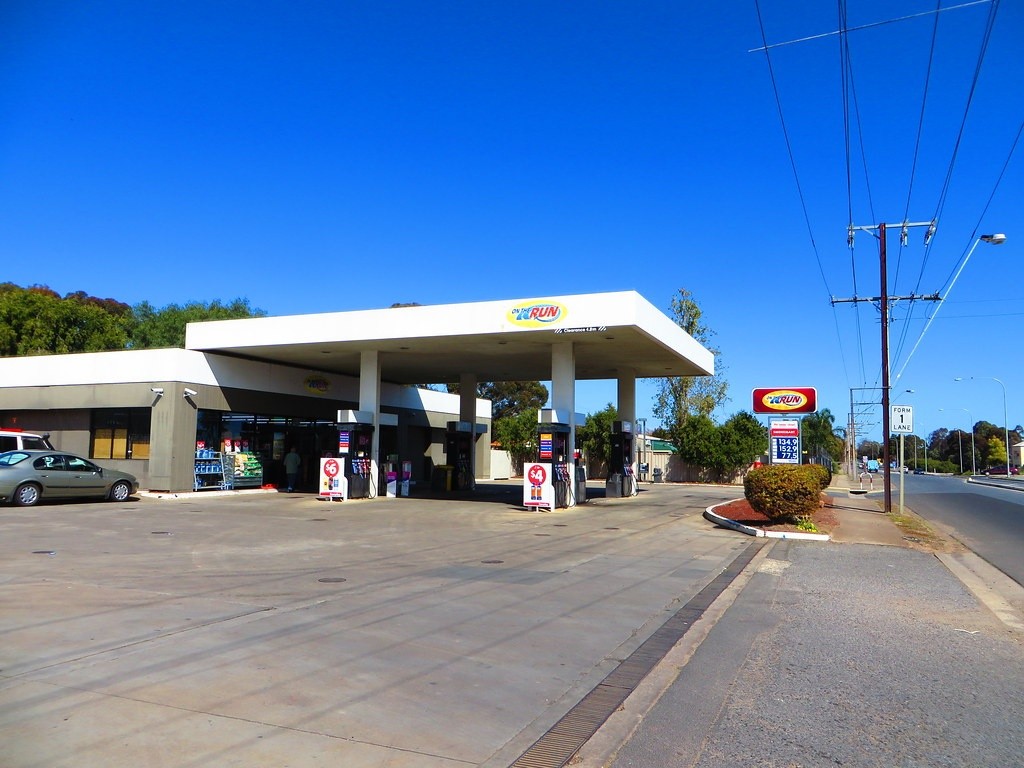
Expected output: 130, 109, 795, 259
954, 376, 1011, 479
882, 233, 1007, 513
938, 407, 976, 475
639, 418, 647, 462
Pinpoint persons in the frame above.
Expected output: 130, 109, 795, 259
283, 447, 300, 492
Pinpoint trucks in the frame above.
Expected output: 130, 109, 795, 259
866, 460, 879, 472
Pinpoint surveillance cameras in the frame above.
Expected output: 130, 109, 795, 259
184, 388, 197, 396
150, 388, 164, 393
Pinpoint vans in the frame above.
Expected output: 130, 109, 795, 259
1, 430, 57, 458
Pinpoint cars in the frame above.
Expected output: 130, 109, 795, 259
858, 463, 865, 469
889, 462, 897, 468
980, 465, 1020, 476
913, 468, 925, 475
1, 449, 140, 507
898, 465, 909, 474
880, 463, 885, 468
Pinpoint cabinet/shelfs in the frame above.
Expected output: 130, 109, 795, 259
193, 451, 226, 491
222, 453, 264, 490
